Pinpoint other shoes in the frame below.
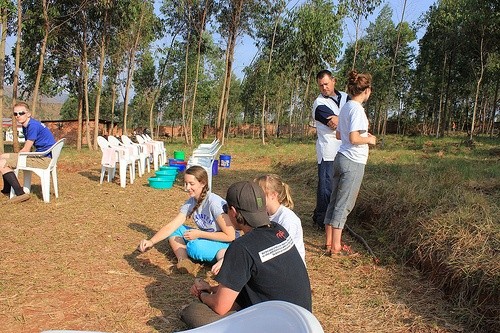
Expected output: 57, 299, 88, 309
325, 243, 351, 255
317, 222, 326, 231
177, 259, 203, 276
0, 190, 10, 199
330, 248, 359, 258
313, 220, 319, 227
8, 194, 31, 203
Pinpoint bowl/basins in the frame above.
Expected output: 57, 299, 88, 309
155, 171, 178, 183
172, 162, 187, 165
170, 163, 186, 172
148, 177, 175, 189
168, 159, 184, 164
159, 167, 178, 172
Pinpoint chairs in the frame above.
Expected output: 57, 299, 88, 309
97, 133, 167, 188
177, 300, 323, 333
10, 138, 67, 202
185, 139, 222, 194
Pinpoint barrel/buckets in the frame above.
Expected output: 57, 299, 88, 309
219, 154, 231, 168
211, 159, 219, 176
174, 150, 186, 161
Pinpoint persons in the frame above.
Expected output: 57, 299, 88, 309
139, 165, 241, 275
180, 180, 313, 327
0, 101, 57, 204
210, 172, 307, 274
312, 69, 350, 231
324, 71, 377, 258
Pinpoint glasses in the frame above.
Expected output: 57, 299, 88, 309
222, 203, 234, 213
13, 110, 29, 116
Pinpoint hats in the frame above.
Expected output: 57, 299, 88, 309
226, 180, 271, 227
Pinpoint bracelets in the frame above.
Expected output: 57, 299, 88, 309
197, 290, 210, 303
149, 239, 153, 244
